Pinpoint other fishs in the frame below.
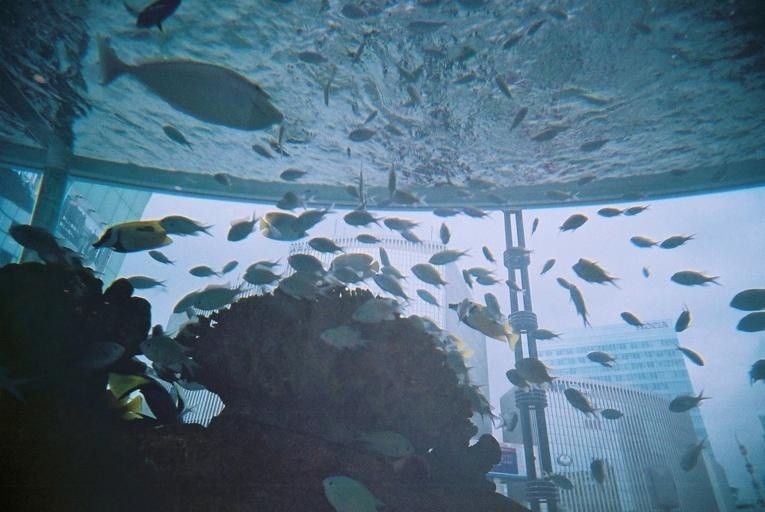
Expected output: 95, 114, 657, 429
730, 288, 764, 333
0, 1, 723, 431
322, 474, 384, 512
353, 427, 416, 460
746, 359, 765, 386
542, 470, 573, 491
680, 434, 708, 471
